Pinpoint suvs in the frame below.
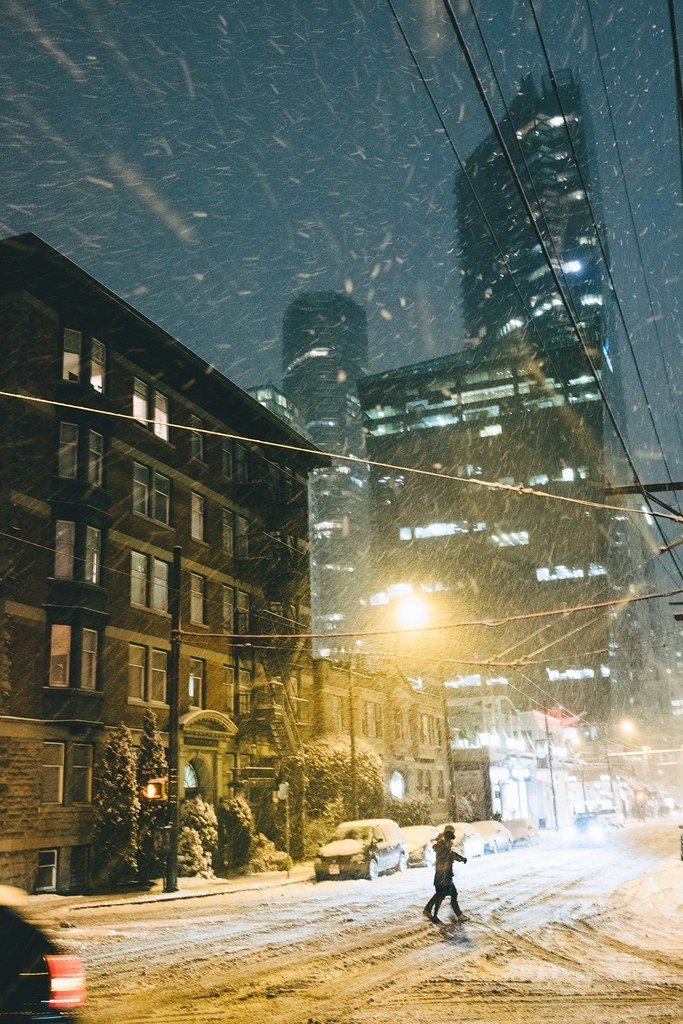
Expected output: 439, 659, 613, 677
313, 820, 407, 879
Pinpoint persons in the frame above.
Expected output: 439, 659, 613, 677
422, 826, 470, 921
430, 830, 462, 925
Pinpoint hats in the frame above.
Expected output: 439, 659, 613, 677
444, 831, 455, 841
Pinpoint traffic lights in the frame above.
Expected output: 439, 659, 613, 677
142, 783, 162, 800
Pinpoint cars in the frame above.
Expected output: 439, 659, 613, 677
502, 818, 538, 848
442, 823, 483, 860
471, 820, 511, 854
401, 826, 440, 867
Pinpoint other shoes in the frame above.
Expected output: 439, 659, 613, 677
457, 915, 469, 921
422, 910, 432, 919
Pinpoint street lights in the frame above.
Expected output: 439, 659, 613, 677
602, 719, 636, 789
271, 767, 290, 880
